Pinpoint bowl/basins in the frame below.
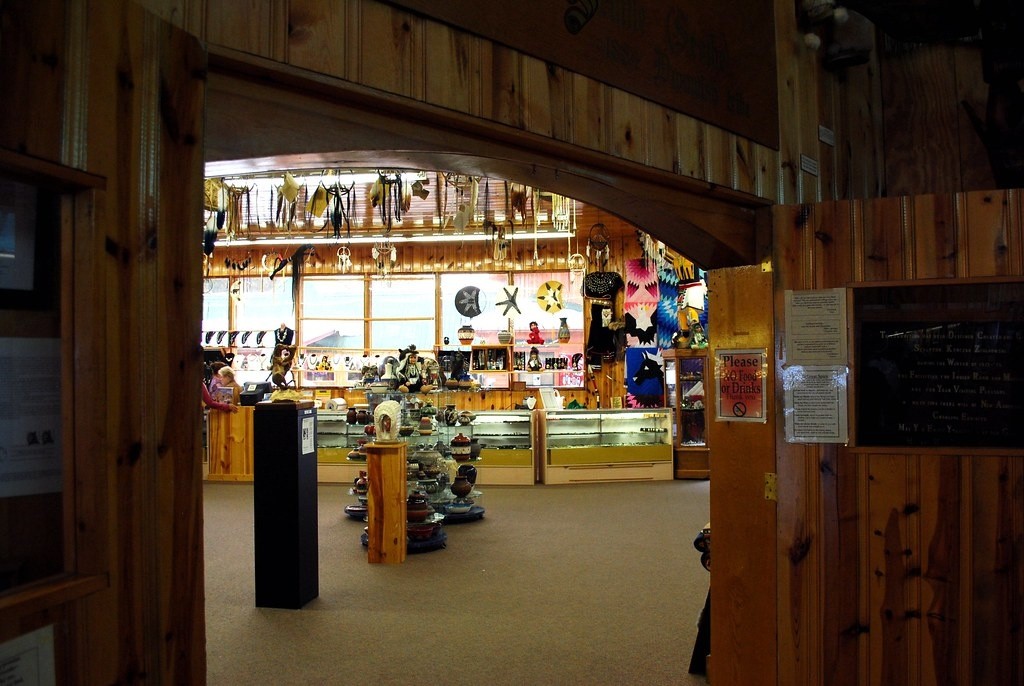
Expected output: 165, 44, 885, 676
407, 525, 433, 540
399, 426, 414, 436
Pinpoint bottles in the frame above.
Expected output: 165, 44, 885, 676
473, 349, 506, 370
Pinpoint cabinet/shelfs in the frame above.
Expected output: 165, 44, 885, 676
367, 393, 442, 502
661, 348, 709, 478
439, 411, 538, 486
540, 408, 675, 484
318, 412, 367, 484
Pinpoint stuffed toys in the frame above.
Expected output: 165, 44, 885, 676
271, 344, 298, 385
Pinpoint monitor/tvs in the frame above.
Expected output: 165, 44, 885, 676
239, 382, 273, 406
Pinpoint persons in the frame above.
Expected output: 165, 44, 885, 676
216, 366, 243, 406
209, 362, 228, 392
275, 322, 293, 346
203, 364, 239, 414
527, 348, 544, 371
396, 345, 430, 393
527, 322, 545, 345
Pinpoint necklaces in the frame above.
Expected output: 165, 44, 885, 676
206, 331, 266, 344
333, 356, 381, 366
234, 354, 268, 368
276, 329, 287, 342
292, 356, 317, 367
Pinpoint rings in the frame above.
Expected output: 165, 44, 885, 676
232, 406, 233, 408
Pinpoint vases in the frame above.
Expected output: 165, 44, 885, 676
558, 318, 570, 343
497, 330, 511, 344
458, 326, 475, 345
